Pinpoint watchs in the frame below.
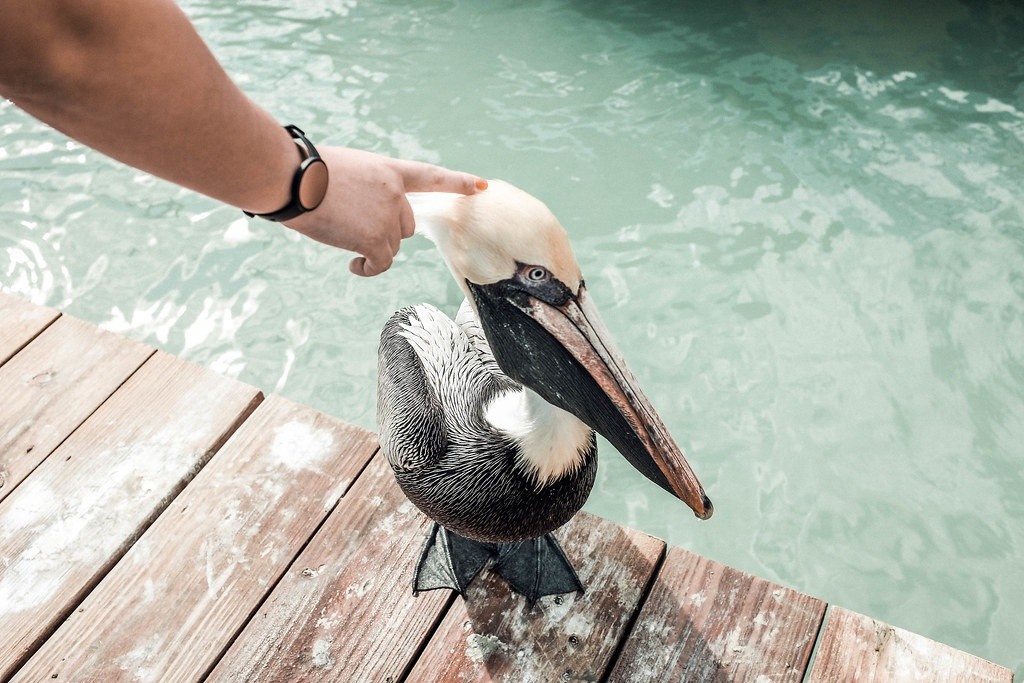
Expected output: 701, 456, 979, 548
243, 124, 331, 224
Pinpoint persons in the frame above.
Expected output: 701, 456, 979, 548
0, 0, 490, 276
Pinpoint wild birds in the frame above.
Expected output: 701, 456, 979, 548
375, 178, 713, 616
